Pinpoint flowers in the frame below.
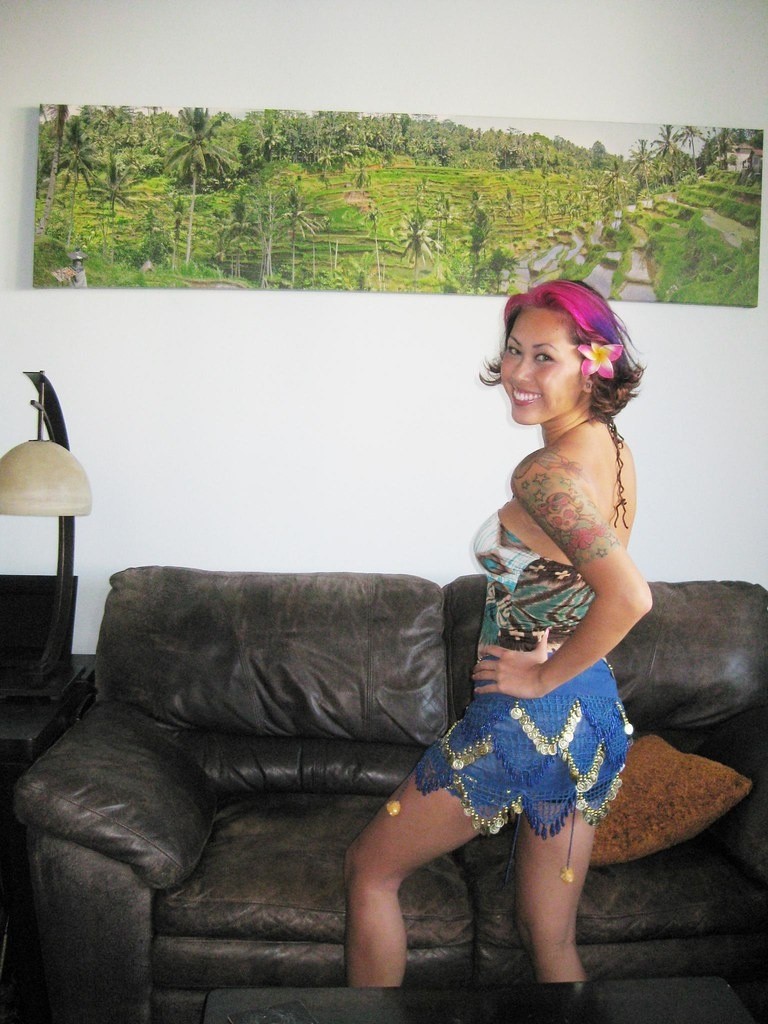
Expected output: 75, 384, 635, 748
578, 343, 624, 379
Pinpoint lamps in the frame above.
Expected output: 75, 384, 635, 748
0, 369, 92, 698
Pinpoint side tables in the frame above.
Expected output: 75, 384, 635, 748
0, 654, 96, 1023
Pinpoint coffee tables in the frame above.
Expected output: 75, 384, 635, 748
203, 976, 763, 1024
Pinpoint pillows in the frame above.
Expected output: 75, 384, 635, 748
590, 731, 751, 867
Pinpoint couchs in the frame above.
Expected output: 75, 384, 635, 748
15, 567, 768, 1024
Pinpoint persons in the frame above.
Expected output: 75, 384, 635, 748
348, 279, 652, 985
